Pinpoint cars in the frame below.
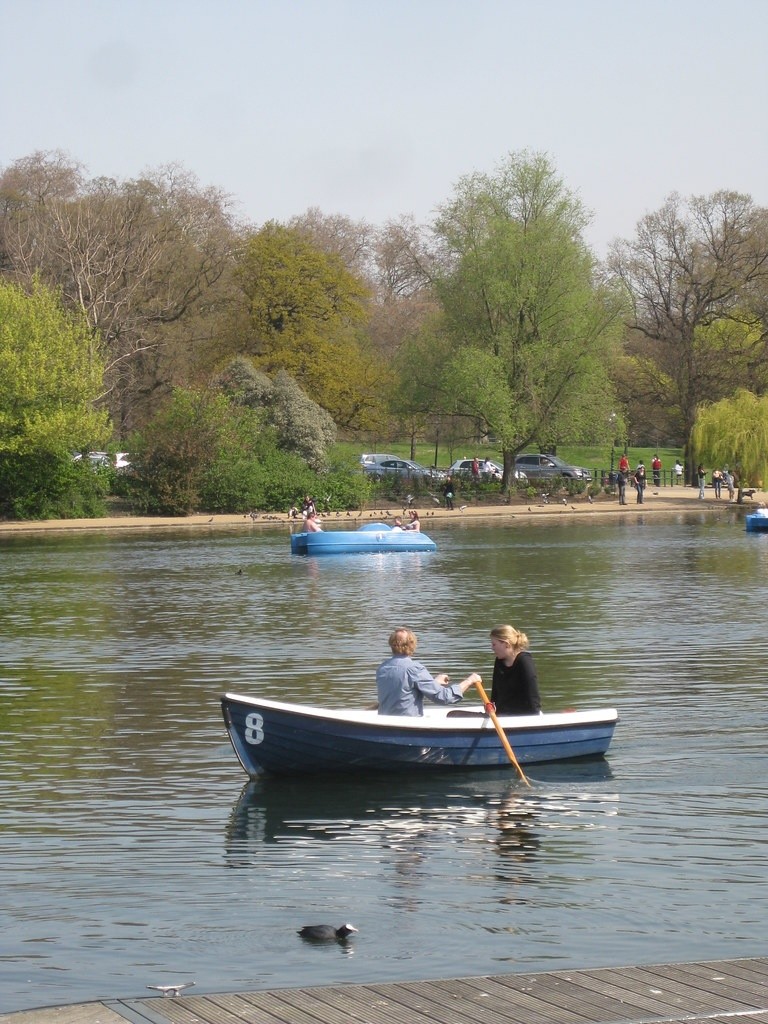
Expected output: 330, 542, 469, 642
359, 453, 400, 468
73, 450, 139, 469
366, 460, 448, 482
448, 458, 529, 482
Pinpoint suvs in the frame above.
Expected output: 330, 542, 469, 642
513, 453, 591, 482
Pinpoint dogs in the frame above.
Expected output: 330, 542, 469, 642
742, 489, 756, 500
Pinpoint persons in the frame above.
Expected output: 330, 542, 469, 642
472, 456, 480, 483
698, 462, 708, 500
650, 454, 662, 487
631, 464, 648, 504
671, 460, 685, 485
618, 454, 630, 482
481, 456, 495, 482
637, 460, 647, 475
376, 628, 482, 717
442, 475, 455, 511
287, 495, 324, 533
393, 511, 420, 532
712, 467, 736, 500
615, 467, 628, 505
446, 624, 543, 718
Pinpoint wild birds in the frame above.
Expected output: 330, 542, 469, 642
243, 490, 659, 520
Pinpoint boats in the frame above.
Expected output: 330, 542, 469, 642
220, 691, 621, 780
288, 521, 436, 555
745, 509, 768, 532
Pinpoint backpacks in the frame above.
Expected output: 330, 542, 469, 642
652, 458, 660, 470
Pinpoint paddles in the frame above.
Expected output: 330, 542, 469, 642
475, 681, 533, 789
363, 678, 450, 710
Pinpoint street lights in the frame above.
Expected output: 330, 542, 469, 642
609, 413, 618, 492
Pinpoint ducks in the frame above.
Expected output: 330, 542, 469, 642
208, 516, 213, 522
298, 923, 360, 940
235, 569, 243, 575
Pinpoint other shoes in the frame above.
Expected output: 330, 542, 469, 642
729, 499, 737, 503
